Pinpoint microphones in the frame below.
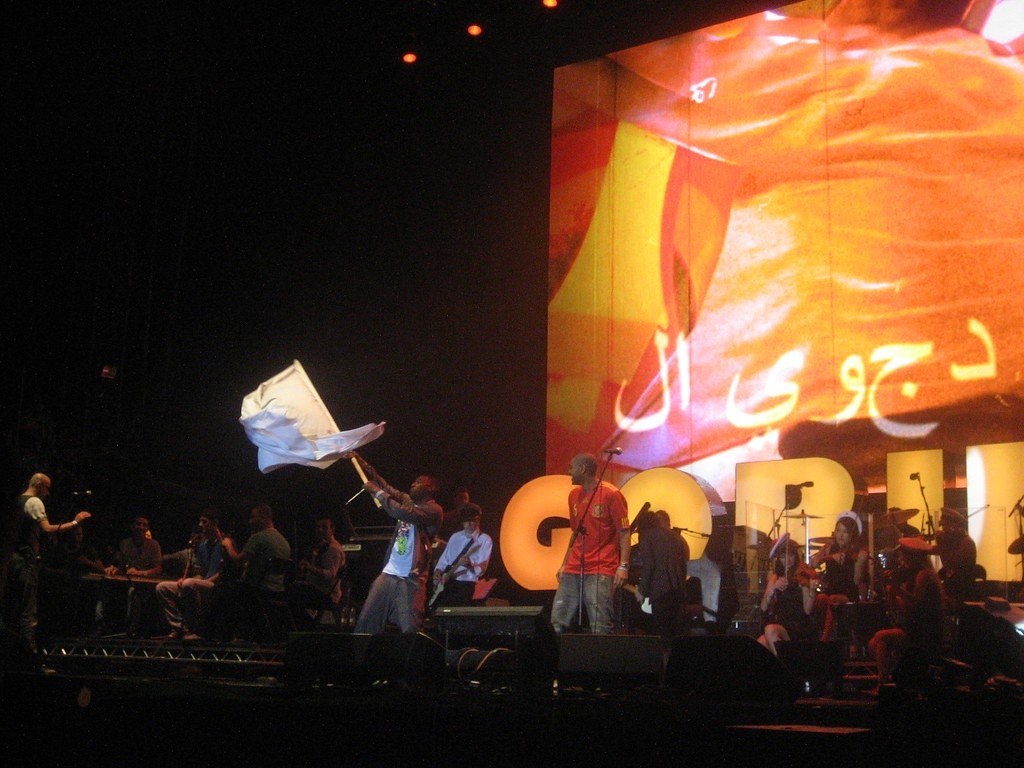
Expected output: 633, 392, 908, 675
603, 447, 622, 455
673, 527, 688, 530
910, 472, 919, 480
73, 491, 91, 494
799, 481, 813, 487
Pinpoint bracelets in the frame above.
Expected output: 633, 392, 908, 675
618, 562, 629, 571
71, 520, 78, 528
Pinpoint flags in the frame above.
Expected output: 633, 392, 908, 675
237, 360, 386, 473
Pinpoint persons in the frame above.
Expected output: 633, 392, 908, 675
429, 502, 493, 605
550, 452, 632, 634
0, 472, 345, 658
634, 506, 976, 697
439, 486, 469, 539
348, 450, 443, 636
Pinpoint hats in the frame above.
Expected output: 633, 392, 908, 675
835, 510, 863, 537
941, 506, 966, 523
898, 536, 934, 553
769, 532, 790, 560
457, 503, 483, 521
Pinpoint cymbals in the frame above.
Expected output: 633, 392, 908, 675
875, 509, 919, 528
813, 536, 835, 544
1008, 535, 1024, 555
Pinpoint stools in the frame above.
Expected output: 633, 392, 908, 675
833, 601, 881, 678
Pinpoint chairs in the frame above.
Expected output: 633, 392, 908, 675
198, 556, 352, 647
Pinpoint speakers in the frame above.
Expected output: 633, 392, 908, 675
556, 631, 800, 726
284, 630, 450, 686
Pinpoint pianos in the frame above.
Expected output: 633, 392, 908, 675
83, 570, 160, 584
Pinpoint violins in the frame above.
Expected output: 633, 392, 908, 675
790, 560, 833, 596
838, 545, 880, 564
300, 538, 329, 575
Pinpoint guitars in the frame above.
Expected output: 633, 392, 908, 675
426, 527, 481, 606
